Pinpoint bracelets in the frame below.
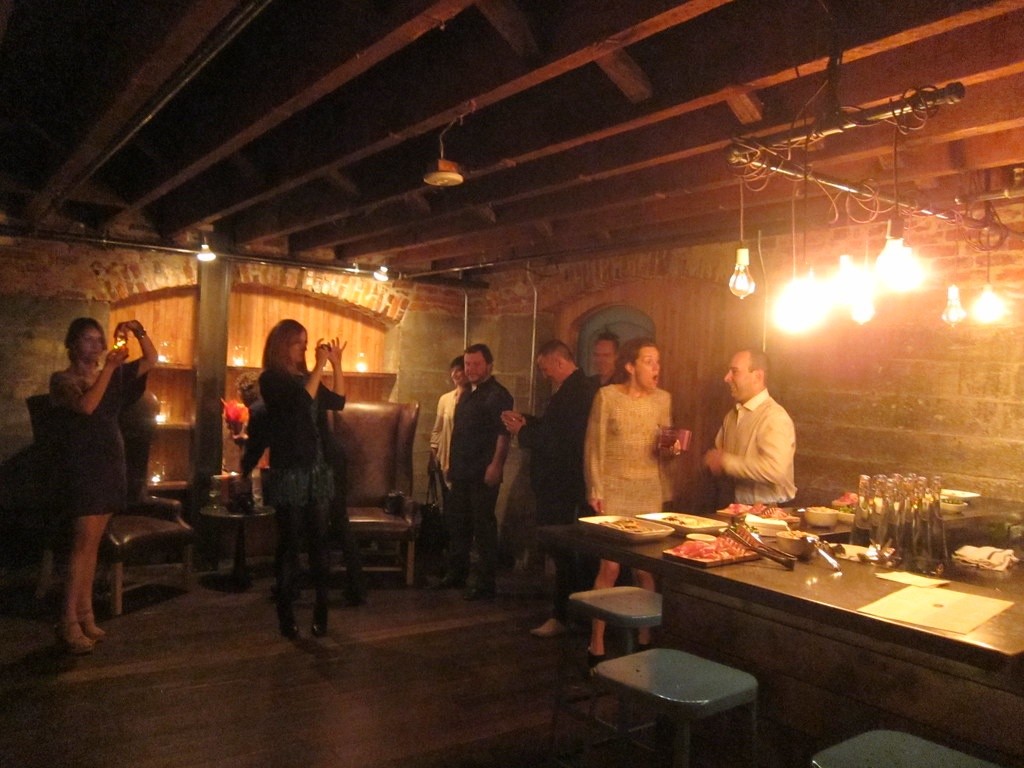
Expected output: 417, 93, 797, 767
135, 330, 147, 340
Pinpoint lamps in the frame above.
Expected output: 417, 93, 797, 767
422, 120, 467, 189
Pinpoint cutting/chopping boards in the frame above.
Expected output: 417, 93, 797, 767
665, 550, 761, 567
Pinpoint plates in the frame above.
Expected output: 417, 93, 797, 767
579, 515, 674, 543
636, 512, 729, 534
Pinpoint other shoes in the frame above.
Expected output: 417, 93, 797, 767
587, 648, 607, 670
462, 573, 485, 600
530, 617, 580, 639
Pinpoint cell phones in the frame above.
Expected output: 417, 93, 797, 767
114, 336, 127, 351
500, 413, 517, 424
321, 344, 332, 352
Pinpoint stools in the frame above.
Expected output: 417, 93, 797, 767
544, 586, 666, 768
583, 647, 758, 768
811, 730, 1002, 768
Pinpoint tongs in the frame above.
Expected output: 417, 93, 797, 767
730, 526, 797, 570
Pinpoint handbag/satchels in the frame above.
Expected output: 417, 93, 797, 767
417, 470, 448, 557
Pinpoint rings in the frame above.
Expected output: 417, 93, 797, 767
674, 452, 681, 456
512, 418, 515, 422
504, 423, 508, 427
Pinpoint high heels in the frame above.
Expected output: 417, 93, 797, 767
310, 598, 329, 636
278, 608, 299, 639
52, 617, 107, 654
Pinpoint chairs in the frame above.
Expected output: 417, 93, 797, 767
326, 400, 421, 588
26, 394, 195, 614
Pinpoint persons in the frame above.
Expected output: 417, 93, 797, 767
238, 319, 371, 637
49, 318, 160, 653
501, 324, 683, 666
704, 349, 796, 510
430, 343, 513, 603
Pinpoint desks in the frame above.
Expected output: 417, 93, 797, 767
197, 505, 274, 589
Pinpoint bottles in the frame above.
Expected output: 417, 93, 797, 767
850, 473, 948, 575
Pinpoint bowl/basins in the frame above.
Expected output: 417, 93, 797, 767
940, 498, 968, 513
778, 530, 820, 557
806, 508, 839, 526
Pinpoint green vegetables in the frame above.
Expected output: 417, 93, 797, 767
839, 504, 868, 515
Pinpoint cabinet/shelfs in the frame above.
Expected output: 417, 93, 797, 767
145, 364, 397, 490
